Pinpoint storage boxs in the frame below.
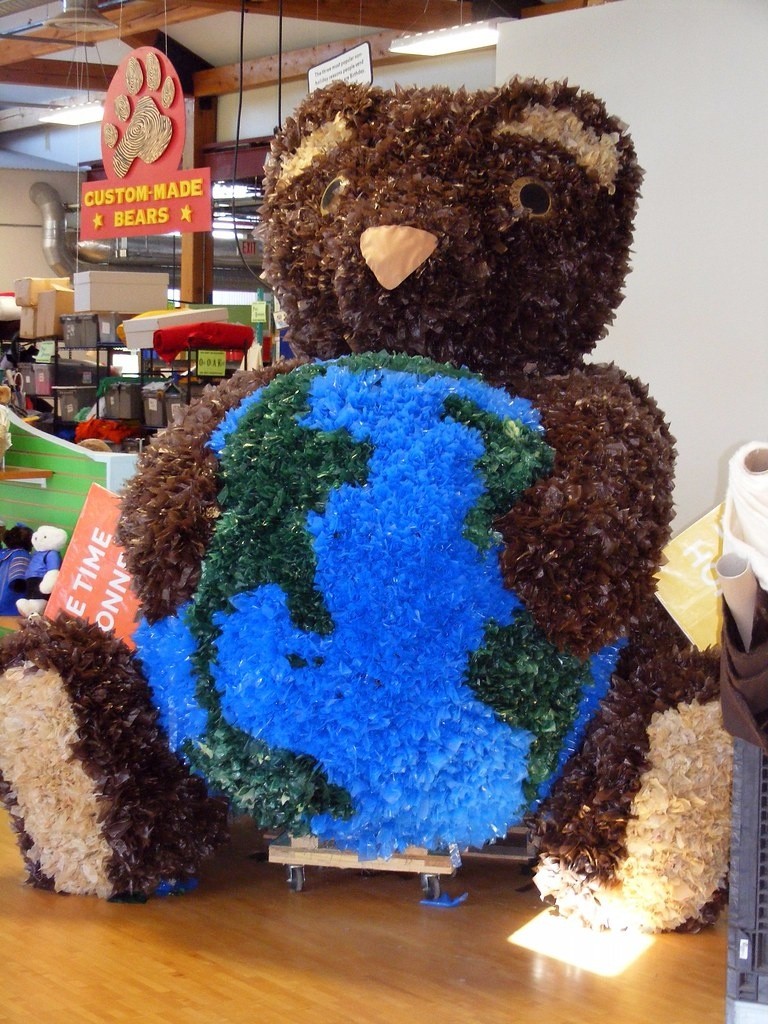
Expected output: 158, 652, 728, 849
14, 270, 229, 429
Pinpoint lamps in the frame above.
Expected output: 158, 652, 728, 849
37, 38, 106, 125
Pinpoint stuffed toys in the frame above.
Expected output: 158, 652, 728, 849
0, 523, 69, 620
0, 76, 738, 934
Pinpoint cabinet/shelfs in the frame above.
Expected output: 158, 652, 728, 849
16, 336, 249, 421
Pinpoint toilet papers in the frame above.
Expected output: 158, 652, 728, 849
718, 437, 768, 592
